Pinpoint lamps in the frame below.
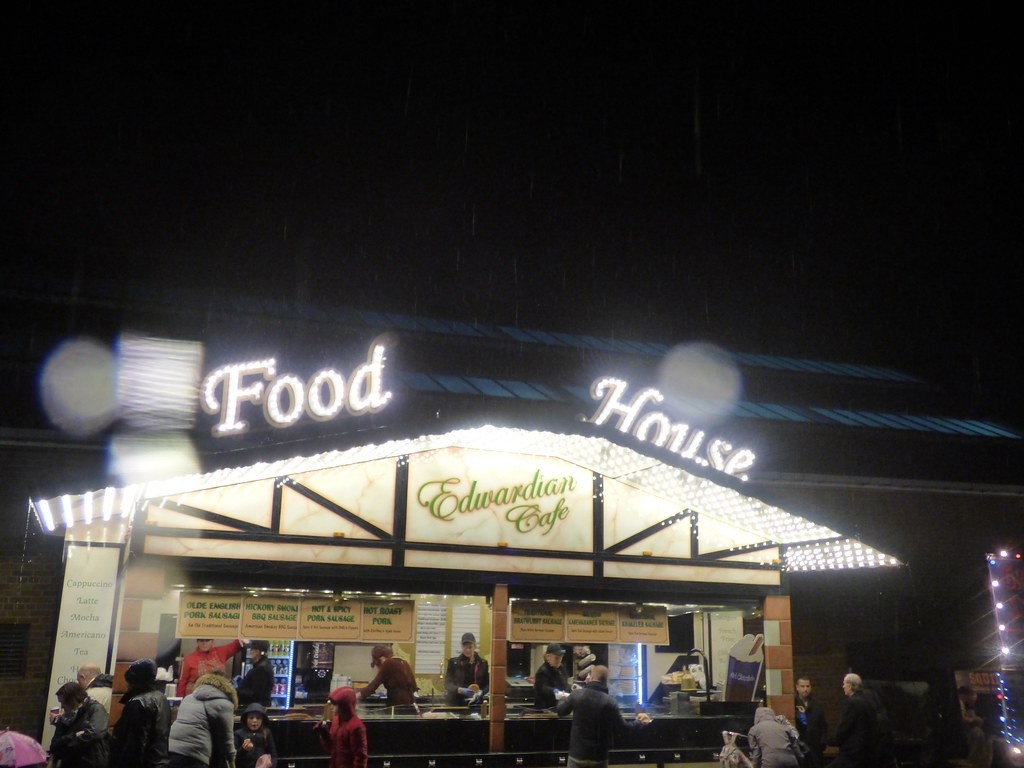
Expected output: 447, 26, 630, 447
332, 590, 343, 602
634, 602, 644, 613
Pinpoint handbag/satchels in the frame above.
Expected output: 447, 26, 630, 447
787, 731, 814, 768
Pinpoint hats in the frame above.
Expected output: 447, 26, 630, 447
244, 639, 270, 652
125, 658, 156, 684
546, 643, 566, 656
462, 633, 476, 646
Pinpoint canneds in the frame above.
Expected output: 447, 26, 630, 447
50, 708, 59, 714
245, 640, 289, 696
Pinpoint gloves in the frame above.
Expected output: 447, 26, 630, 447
553, 689, 559, 694
473, 690, 483, 704
462, 688, 474, 697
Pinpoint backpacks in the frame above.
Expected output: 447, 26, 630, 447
719, 731, 756, 767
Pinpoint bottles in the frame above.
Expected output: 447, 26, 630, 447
168, 665, 174, 681
244, 640, 292, 708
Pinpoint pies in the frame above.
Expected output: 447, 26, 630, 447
469, 684, 479, 693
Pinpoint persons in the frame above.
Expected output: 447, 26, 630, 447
47, 638, 276, 768
355, 645, 421, 715
557, 665, 644, 768
938, 686, 985, 761
445, 632, 489, 714
794, 675, 828, 768
525, 643, 573, 714
747, 707, 804, 768
313, 686, 368, 768
826, 673, 879, 768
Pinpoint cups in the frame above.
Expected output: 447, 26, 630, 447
50, 707, 60, 714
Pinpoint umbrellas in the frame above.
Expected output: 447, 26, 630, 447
0, 726, 50, 768
